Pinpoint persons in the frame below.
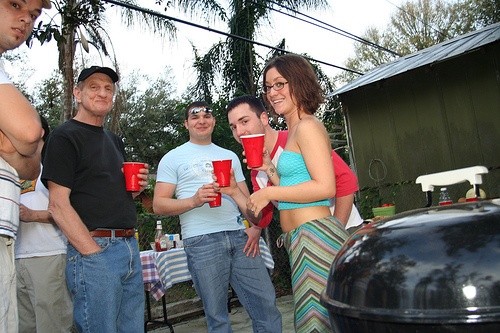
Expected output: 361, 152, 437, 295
0, 0, 52, 333
212, 95, 364, 236
153, 101, 282, 333
40, 66, 149, 333
15, 116, 73, 333
246, 55, 350, 333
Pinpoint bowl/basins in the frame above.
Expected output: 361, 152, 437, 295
150, 241, 173, 250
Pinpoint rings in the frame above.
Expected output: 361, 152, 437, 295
251, 209, 254, 212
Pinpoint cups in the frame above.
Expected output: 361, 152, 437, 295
122, 162, 145, 192
466, 198, 480, 201
439, 201, 453, 205
240, 134, 266, 168
206, 183, 222, 208
211, 159, 232, 187
371, 206, 396, 216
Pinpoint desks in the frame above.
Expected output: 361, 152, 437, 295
139, 236, 275, 333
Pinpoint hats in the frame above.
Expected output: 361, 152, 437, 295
77, 66, 118, 84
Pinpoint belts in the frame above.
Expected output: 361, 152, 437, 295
89, 228, 135, 237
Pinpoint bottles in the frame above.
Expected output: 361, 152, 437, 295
155, 220, 167, 251
439, 187, 451, 202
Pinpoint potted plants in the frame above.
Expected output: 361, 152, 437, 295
372, 203, 396, 217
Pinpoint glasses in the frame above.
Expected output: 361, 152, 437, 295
188, 107, 213, 114
262, 81, 289, 94
